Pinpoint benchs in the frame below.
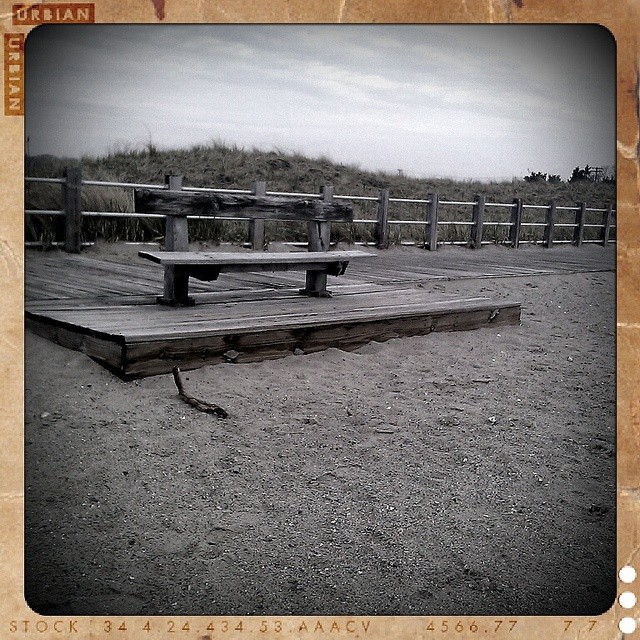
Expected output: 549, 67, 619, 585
138, 249, 377, 309
